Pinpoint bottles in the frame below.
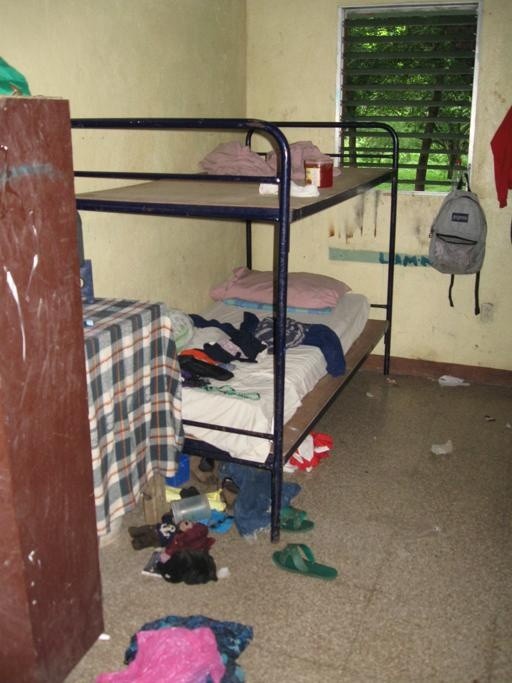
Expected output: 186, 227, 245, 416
170, 493, 213, 526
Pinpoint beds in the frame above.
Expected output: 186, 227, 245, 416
70, 117, 400, 541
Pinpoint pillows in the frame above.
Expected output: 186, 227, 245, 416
211, 266, 350, 309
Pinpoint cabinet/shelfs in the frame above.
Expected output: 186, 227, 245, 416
1, 98, 103, 683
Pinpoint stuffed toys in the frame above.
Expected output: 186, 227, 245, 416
129, 509, 193, 555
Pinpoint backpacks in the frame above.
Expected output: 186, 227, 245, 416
428, 174, 487, 274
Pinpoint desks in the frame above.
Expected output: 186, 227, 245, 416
83, 297, 185, 552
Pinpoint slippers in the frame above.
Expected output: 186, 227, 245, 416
279, 507, 315, 532
272, 543, 337, 580
